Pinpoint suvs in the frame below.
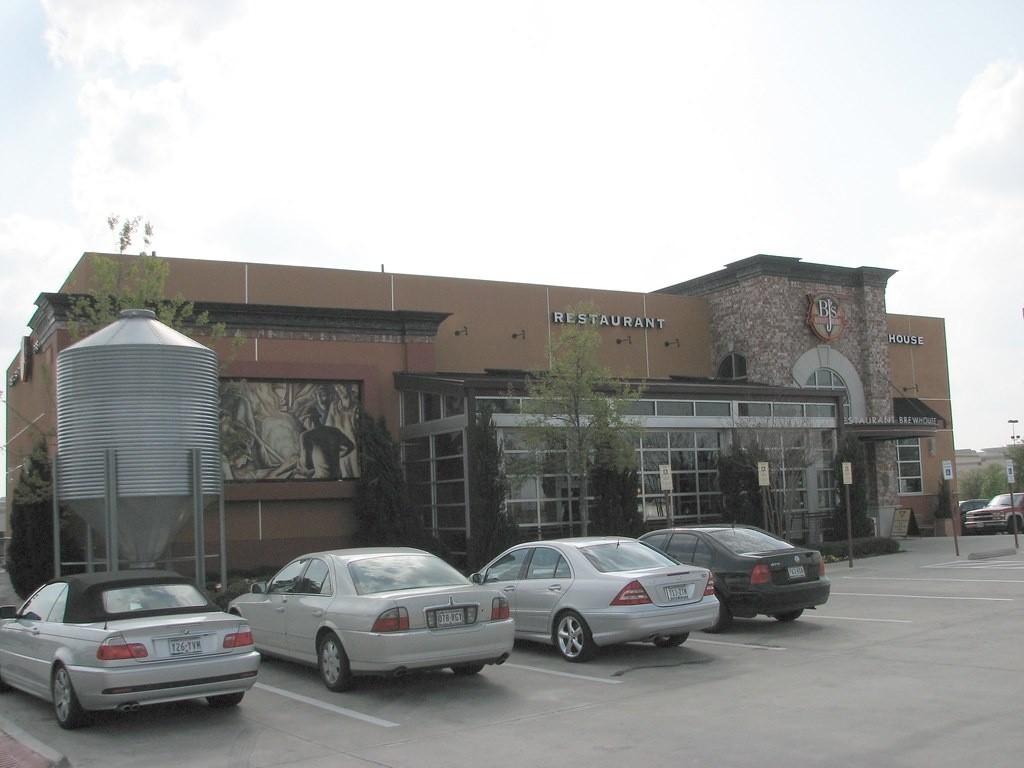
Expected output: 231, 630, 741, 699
963, 492, 1024, 535
959, 499, 991, 523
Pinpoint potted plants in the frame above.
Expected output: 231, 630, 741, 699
930, 471, 962, 537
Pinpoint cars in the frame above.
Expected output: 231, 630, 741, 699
636, 523, 832, 634
227, 545, 515, 693
466, 536, 721, 664
0, 569, 261, 731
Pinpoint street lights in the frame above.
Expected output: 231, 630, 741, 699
1007, 418, 1020, 446
